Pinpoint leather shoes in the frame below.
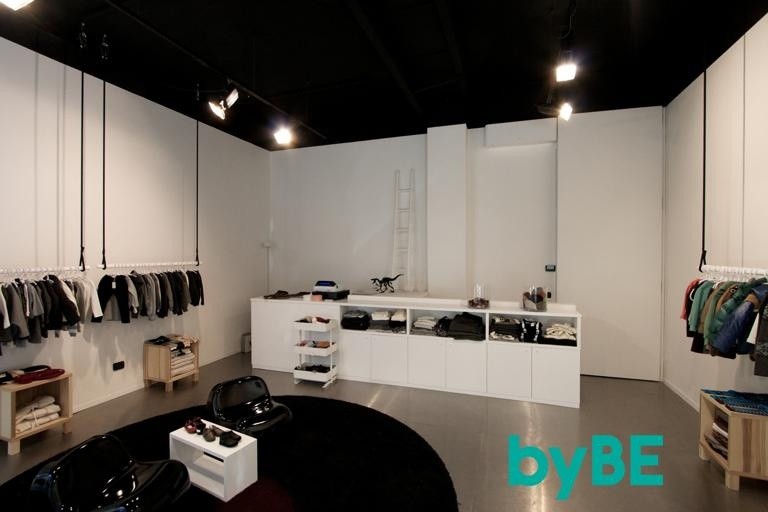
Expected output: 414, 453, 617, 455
185, 416, 241, 447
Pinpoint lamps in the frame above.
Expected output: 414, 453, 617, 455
208, 81, 240, 124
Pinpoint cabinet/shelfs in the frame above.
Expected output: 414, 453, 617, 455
0, 367, 74, 456
142, 336, 199, 392
250, 300, 582, 408
699, 390, 768, 492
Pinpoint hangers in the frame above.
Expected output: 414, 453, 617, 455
0, 260, 200, 289
697, 265, 768, 289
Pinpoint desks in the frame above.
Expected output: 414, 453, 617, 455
0, 394, 459, 511
